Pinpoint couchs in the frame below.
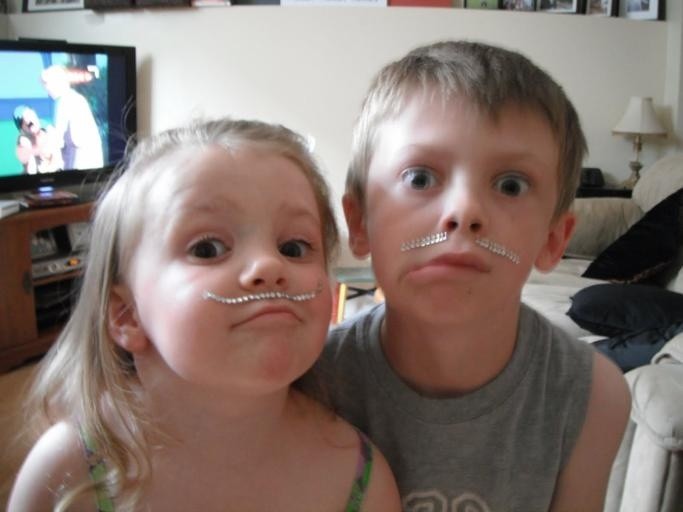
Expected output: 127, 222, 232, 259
520, 150, 683, 511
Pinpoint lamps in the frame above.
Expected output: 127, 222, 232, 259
609, 94, 666, 188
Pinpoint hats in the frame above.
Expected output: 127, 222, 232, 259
11, 105, 30, 130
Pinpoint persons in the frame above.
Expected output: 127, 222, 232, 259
36, 63, 103, 170
10, 105, 63, 176
2, 117, 404, 512
290, 40, 631, 511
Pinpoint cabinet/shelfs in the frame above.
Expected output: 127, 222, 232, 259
0, 192, 103, 372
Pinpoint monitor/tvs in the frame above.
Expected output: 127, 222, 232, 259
0, 40, 138, 192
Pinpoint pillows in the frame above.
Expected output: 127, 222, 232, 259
564, 185, 682, 374
